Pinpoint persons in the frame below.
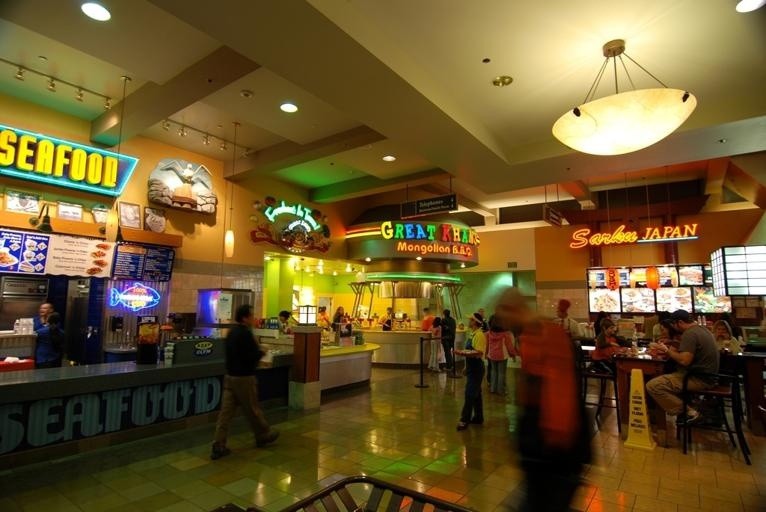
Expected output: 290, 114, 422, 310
427, 317, 442, 373
32, 302, 54, 332
378, 307, 408, 331
493, 286, 591, 512
452, 299, 746, 432
438, 309, 456, 371
333, 306, 345, 331
421, 307, 435, 330
317, 307, 330, 328
78, 325, 102, 365
278, 311, 292, 335
211, 304, 279, 460
33, 312, 65, 369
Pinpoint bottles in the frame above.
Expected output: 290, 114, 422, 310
697, 314, 707, 326
631, 333, 638, 347
270, 317, 279, 329
14, 317, 33, 335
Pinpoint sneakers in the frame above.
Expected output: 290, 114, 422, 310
456, 421, 468, 431
674, 406, 704, 427
255, 431, 281, 448
443, 366, 452, 371
432, 367, 442, 372
470, 417, 484, 424
210, 447, 232, 459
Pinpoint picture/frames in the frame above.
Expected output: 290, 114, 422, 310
1, 185, 43, 214
53, 200, 84, 223
116, 199, 143, 232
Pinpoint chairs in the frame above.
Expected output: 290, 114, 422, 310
568, 330, 766, 467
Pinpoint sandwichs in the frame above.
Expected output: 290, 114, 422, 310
19, 262, 35, 274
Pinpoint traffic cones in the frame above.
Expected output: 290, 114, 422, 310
621, 366, 658, 454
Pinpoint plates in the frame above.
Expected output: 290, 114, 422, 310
455, 347, 483, 355
86, 243, 112, 275
0, 239, 48, 272
589, 288, 692, 312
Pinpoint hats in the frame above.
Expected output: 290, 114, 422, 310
663, 309, 690, 323
465, 312, 483, 324
557, 299, 571, 312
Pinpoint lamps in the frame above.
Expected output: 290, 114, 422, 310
580, 167, 680, 295
159, 117, 234, 151
550, 37, 697, 156
223, 121, 240, 259
0, 56, 111, 112
